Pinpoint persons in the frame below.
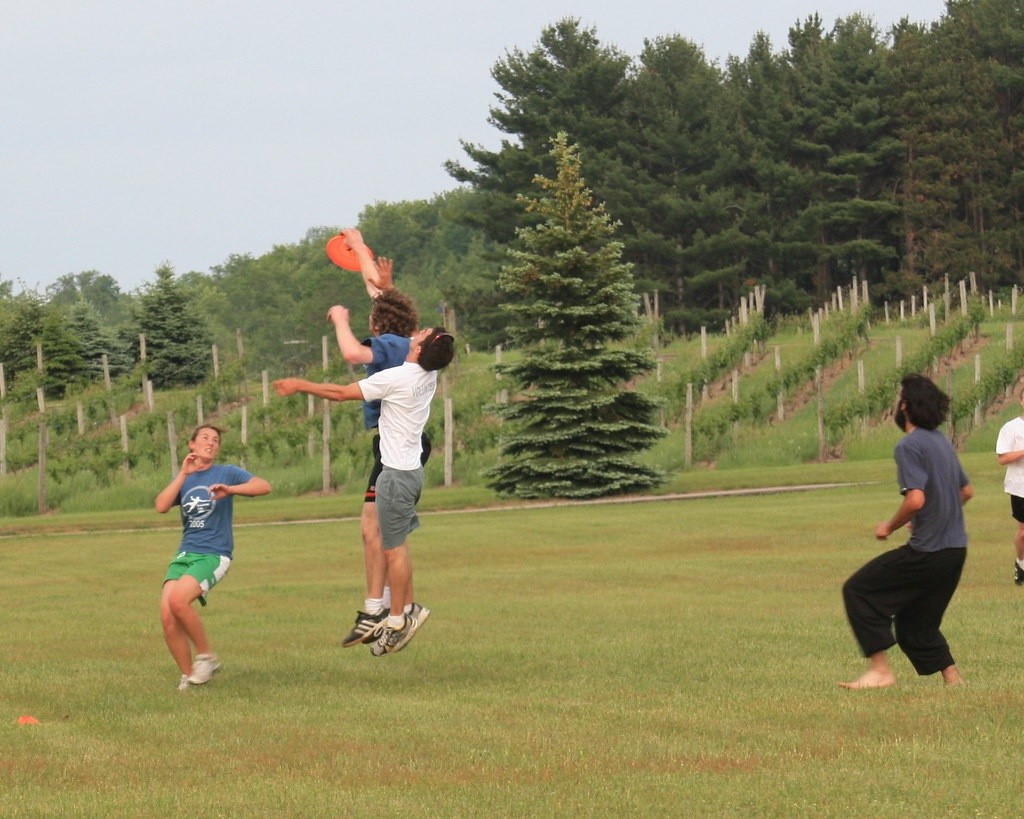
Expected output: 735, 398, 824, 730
834, 372, 976, 690
996, 387, 1024, 586
271, 256, 454, 657
154, 423, 272, 691
326, 228, 431, 648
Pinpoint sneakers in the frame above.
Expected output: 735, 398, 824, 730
361, 625, 385, 644
176, 674, 190, 690
369, 612, 417, 657
188, 654, 221, 685
342, 604, 390, 647
409, 603, 430, 628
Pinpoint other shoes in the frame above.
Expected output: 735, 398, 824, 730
1015, 562, 1024, 585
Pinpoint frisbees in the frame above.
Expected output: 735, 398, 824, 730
326, 235, 375, 272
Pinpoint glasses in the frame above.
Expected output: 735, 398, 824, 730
431, 333, 454, 344
